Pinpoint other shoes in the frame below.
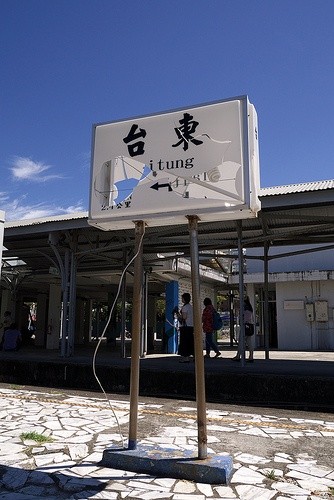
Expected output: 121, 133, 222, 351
214, 353, 221, 357
178, 356, 190, 362
204, 355, 210, 359
231, 357, 240, 362
246, 359, 253, 362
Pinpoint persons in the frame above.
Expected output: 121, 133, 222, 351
202, 297, 220, 358
1, 323, 22, 352
175, 292, 195, 362
233, 295, 255, 360
0, 311, 16, 344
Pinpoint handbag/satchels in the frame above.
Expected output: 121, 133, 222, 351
244, 321, 254, 337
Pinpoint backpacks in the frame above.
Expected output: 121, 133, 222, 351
209, 308, 222, 330
